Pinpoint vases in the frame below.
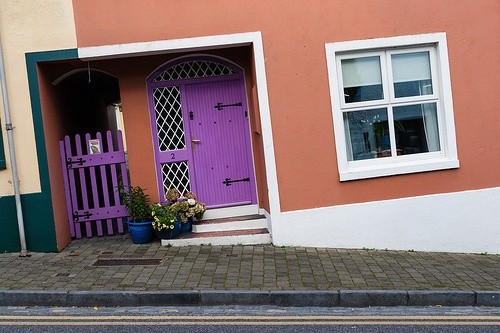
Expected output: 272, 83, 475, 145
178, 220, 192, 235
156, 221, 183, 239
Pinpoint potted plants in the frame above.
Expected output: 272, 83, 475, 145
114, 181, 155, 244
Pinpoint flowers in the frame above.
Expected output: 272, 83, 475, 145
150, 202, 184, 231
165, 188, 207, 224
